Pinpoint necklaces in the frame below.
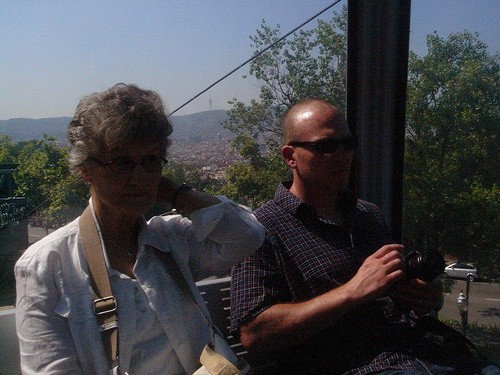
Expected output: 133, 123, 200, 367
126, 248, 133, 256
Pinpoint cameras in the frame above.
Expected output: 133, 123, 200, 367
393, 247, 445, 284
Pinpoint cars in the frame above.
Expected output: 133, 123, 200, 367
440, 261, 481, 282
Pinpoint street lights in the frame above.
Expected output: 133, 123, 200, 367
456, 275, 471, 337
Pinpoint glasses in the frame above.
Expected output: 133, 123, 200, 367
88, 154, 169, 175
287, 136, 359, 157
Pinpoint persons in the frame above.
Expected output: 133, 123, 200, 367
14, 85, 265, 375
229, 98, 500, 375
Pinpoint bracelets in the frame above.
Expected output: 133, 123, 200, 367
171, 183, 193, 208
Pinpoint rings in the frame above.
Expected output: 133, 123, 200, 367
418, 299, 424, 305
